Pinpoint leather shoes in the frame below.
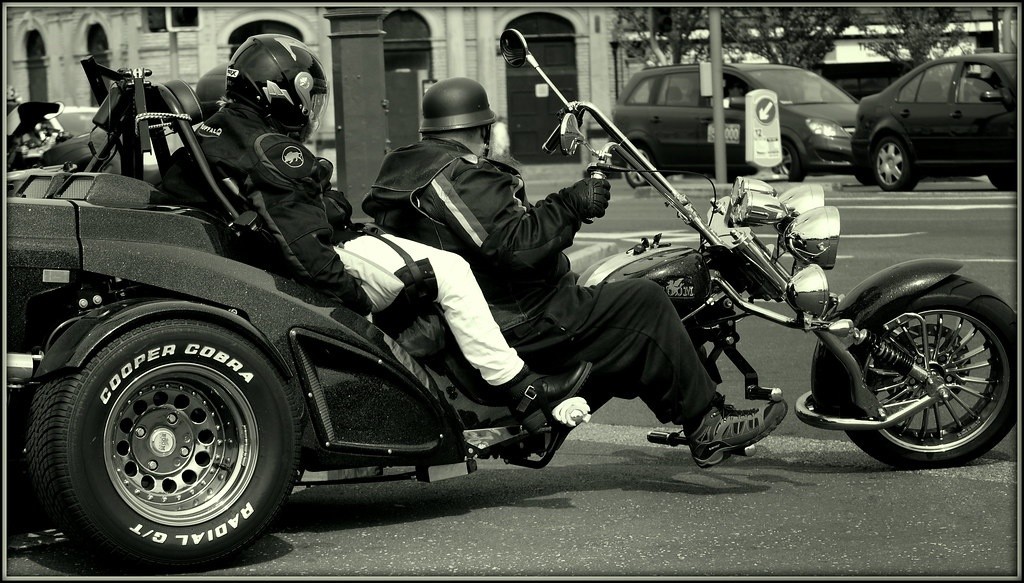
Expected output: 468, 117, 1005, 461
505, 358, 594, 435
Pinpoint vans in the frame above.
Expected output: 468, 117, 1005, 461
606, 62, 862, 189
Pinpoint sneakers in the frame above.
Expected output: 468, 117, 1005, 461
682, 392, 789, 472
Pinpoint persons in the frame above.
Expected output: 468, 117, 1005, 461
361, 94, 790, 468
159, 46, 594, 434
726, 80, 746, 98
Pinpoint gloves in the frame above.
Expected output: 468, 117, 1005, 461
573, 176, 612, 221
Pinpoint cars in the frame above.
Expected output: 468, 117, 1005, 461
850, 51, 1018, 193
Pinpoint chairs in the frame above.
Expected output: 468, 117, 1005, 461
84, 61, 441, 325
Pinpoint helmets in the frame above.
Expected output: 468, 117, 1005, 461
418, 77, 497, 136
196, 64, 225, 121
225, 33, 330, 147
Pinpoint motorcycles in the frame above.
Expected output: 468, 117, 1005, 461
6, 26, 1018, 570
